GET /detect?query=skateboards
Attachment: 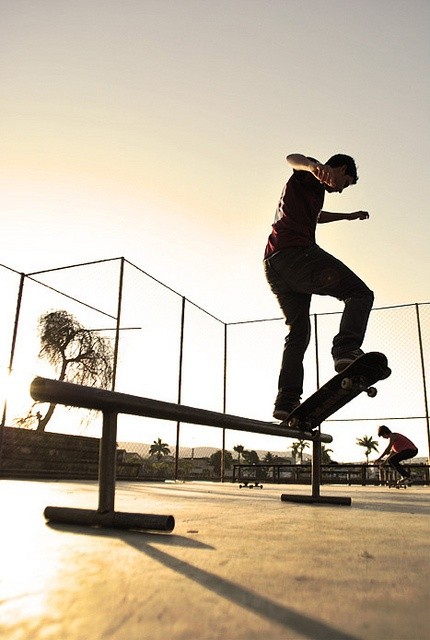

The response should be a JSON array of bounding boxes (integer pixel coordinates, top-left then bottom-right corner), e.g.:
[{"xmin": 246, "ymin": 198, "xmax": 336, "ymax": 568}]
[
  {"xmin": 388, "ymin": 481, "xmax": 412, "ymax": 487},
  {"xmin": 236, "ymin": 478, "xmax": 263, "ymax": 489},
  {"xmin": 280, "ymin": 351, "xmax": 391, "ymax": 441}
]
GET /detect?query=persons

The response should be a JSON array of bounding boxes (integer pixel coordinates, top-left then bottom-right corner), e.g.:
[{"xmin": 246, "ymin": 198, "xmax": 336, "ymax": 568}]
[
  {"xmin": 377, "ymin": 425, "xmax": 418, "ymax": 481},
  {"xmin": 264, "ymin": 154, "xmax": 375, "ymax": 420}
]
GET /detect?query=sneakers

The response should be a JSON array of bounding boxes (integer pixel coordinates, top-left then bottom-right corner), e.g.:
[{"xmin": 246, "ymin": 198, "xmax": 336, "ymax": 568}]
[
  {"xmin": 403, "ymin": 476, "xmax": 413, "ymax": 483},
  {"xmin": 396, "ymin": 475, "xmax": 407, "ymax": 483},
  {"xmin": 272, "ymin": 397, "xmax": 300, "ymax": 419},
  {"xmin": 333, "ymin": 348, "xmax": 363, "ymax": 372}
]
